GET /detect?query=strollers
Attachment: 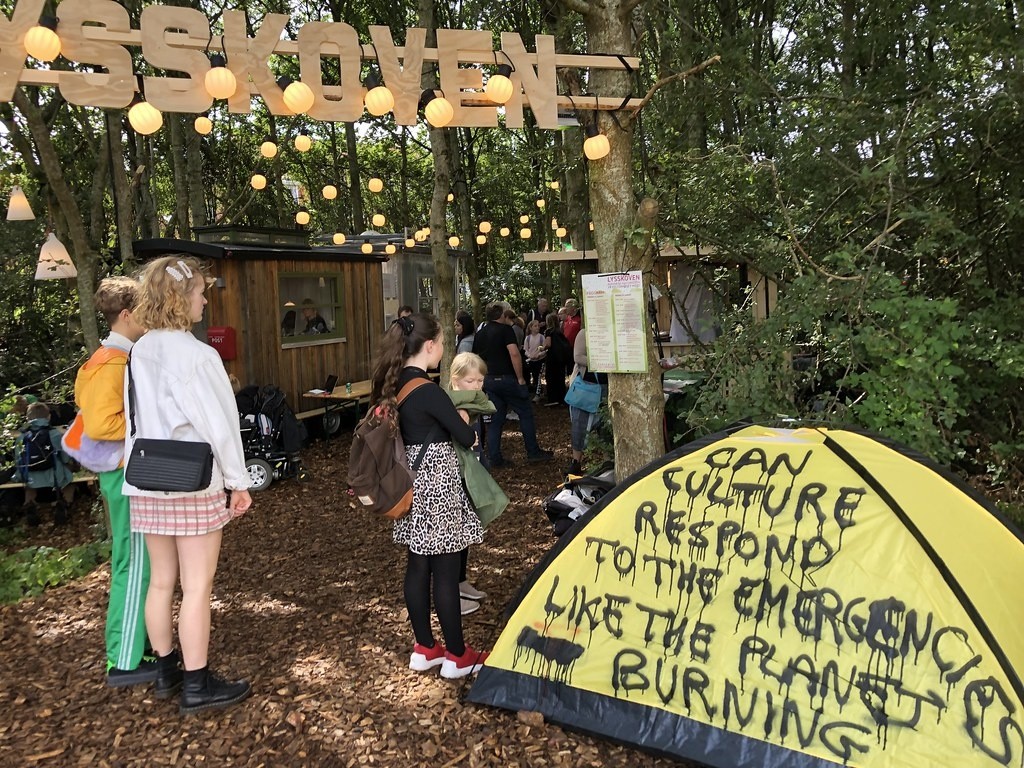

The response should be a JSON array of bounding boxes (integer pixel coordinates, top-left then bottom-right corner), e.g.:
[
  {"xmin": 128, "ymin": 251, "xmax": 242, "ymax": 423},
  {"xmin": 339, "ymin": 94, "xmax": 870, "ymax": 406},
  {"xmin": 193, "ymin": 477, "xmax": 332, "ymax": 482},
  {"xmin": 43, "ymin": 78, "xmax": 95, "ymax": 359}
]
[{"xmin": 233, "ymin": 384, "xmax": 305, "ymax": 492}]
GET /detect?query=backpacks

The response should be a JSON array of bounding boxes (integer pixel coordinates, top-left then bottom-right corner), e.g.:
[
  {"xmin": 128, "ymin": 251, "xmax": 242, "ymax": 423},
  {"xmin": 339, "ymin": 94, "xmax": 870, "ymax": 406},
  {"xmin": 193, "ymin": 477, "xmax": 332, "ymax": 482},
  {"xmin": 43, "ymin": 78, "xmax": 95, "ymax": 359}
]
[
  {"xmin": 346, "ymin": 377, "xmax": 436, "ymax": 520},
  {"xmin": 20, "ymin": 426, "xmax": 56, "ymax": 472},
  {"xmin": 551, "ymin": 332, "xmax": 573, "ymax": 362}
]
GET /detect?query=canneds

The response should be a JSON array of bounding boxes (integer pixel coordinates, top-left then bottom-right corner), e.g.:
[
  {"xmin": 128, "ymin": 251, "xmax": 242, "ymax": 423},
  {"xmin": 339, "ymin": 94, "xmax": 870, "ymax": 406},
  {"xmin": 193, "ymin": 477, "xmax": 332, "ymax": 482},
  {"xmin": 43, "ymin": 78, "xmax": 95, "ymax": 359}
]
[{"xmin": 346, "ymin": 383, "xmax": 351, "ymax": 394}]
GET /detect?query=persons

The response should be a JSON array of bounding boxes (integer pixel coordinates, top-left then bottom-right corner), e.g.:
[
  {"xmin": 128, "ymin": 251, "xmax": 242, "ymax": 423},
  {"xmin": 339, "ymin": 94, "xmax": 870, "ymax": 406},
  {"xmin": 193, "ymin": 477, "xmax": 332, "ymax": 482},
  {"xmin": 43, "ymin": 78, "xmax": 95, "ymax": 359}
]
[
  {"xmin": 455, "ymin": 297, "xmax": 583, "ymax": 423},
  {"xmin": 367, "ymin": 313, "xmax": 493, "ymax": 678},
  {"xmin": 568, "ymin": 328, "xmax": 608, "ymax": 476},
  {"xmin": 124, "ymin": 254, "xmax": 253, "ymax": 712},
  {"xmin": 282, "ymin": 298, "xmax": 331, "ymax": 338},
  {"xmin": 12, "ymin": 394, "xmax": 81, "ymax": 527},
  {"xmin": 445, "ymin": 349, "xmax": 489, "ymax": 616},
  {"xmin": 74, "ymin": 275, "xmax": 159, "ymax": 689},
  {"xmin": 398, "ymin": 306, "xmax": 414, "ymax": 319},
  {"xmin": 472, "ymin": 305, "xmax": 554, "ymax": 468}
]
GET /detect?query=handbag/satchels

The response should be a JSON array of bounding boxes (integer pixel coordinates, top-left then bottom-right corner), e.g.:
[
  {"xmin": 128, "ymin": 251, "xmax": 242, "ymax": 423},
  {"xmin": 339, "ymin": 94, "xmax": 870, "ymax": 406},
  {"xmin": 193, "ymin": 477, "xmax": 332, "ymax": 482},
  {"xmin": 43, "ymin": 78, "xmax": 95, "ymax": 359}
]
[
  {"xmin": 125, "ymin": 343, "xmax": 215, "ymax": 492},
  {"xmin": 61, "ymin": 409, "xmax": 127, "ymax": 474},
  {"xmin": 564, "ymin": 372, "xmax": 602, "ymax": 414}
]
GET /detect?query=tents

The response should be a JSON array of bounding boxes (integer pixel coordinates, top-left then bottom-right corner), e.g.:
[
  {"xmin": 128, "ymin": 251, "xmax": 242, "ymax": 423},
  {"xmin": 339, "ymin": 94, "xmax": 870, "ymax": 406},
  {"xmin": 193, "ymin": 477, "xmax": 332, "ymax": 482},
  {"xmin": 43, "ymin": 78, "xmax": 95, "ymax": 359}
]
[{"xmin": 460, "ymin": 417, "xmax": 1024, "ymax": 768}]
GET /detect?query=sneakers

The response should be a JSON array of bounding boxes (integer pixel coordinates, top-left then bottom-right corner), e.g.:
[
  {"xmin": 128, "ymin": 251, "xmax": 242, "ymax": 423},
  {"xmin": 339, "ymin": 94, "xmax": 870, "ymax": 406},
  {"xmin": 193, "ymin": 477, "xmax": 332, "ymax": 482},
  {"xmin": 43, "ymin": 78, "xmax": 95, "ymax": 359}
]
[
  {"xmin": 532, "ymin": 392, "xmax": 545, "ymax": 403},
  {"xmin": 409, "ymin": 639, "xmax": 445, "ymax": 672},
  {"xmin": 460, "ymin": 579, "xmax": 487, "ymax": 600},
  {"xmin": 440, "ymin": 645, "xmax": 491, "ymax": 679},
  {"xmin": 460, "ymin": 598, "xmax": 480, "ymax": 615},
  {"xmin": 109, "ymin": 656, "xmax": 182, "ymax": 687}
]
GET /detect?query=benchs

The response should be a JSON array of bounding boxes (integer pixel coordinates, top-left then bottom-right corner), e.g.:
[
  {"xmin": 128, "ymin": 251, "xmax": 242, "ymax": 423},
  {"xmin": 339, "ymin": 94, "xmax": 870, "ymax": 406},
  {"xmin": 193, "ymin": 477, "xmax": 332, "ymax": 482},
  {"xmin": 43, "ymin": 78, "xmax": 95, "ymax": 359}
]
[{"xmin": 0, "ymin": 471, "xmax": 98, "ymax": 504}]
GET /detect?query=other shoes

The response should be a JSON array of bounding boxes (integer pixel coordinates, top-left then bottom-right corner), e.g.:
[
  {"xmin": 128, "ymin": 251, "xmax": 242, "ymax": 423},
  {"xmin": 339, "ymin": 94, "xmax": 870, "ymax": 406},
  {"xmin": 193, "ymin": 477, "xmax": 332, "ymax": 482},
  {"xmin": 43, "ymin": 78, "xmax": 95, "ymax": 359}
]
[
  {"xmin": 488, "ymin": 458, "xmax": 515, "ymax": 468},
  {"xmin": 528, "ymin": 450, "xmax": 554, "ymax": 461}
]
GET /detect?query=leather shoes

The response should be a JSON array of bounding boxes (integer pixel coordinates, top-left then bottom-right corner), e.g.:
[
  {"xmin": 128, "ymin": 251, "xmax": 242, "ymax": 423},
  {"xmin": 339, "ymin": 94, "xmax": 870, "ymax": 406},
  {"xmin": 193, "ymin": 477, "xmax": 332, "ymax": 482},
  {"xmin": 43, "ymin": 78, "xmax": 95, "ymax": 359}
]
[
  {"xmin": 179, "ymin": 674, "xmax": 252, "ymax": 714},
  {"xmin": 156, "ymin": 666, "xmax": 183, "ymax": 700}
]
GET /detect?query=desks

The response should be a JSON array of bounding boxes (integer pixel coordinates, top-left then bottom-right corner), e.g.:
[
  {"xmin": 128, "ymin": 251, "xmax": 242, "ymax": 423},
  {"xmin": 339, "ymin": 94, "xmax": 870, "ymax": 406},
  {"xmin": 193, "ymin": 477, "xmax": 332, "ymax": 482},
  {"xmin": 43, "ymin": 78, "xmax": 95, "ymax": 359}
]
[{"xmin": 303, "ymin": 372, "xmax": 441, "ymax": 448}]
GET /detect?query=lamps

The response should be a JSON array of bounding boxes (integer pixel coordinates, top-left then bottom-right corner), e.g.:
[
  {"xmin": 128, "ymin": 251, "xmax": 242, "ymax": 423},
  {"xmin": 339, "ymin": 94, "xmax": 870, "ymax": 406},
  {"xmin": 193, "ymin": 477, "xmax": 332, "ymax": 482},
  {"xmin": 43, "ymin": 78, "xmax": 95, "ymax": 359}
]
[
  {"xmin": 6, "ymin": 119, "xmax": 37, "ymax": 221},
  {"xmin": 34, "ymin": 196, "xmax": 78, "ymax": 278}
]
